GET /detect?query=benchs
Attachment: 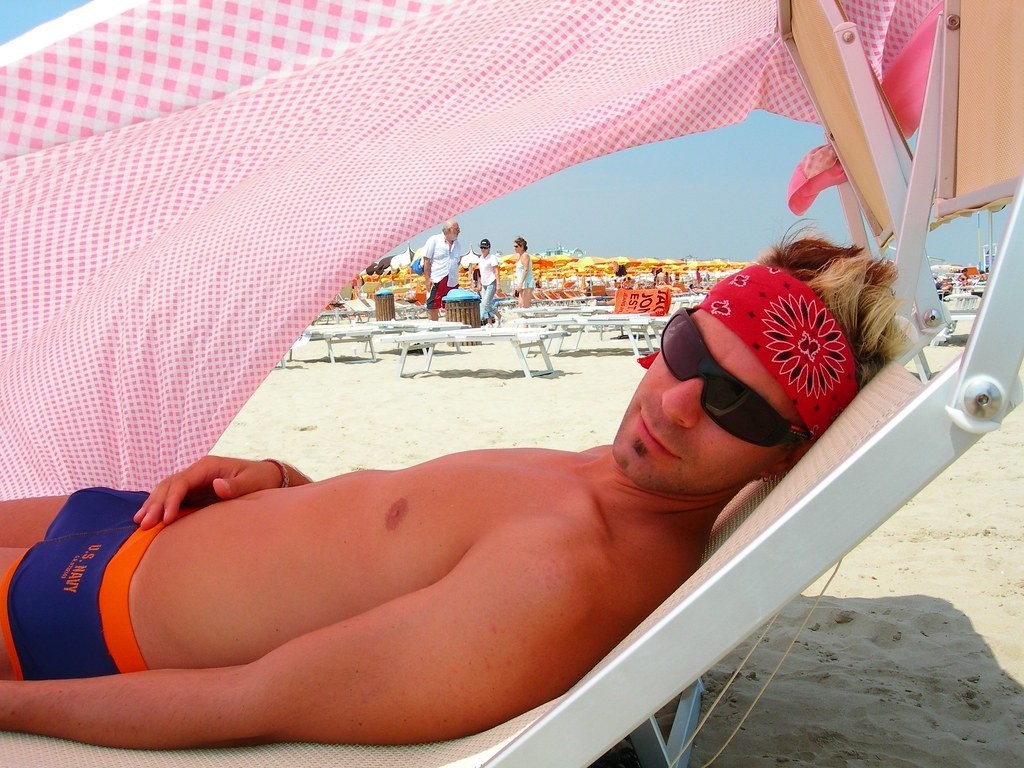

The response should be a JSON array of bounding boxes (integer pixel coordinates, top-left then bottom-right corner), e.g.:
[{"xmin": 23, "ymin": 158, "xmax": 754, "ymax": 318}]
[{"xmin": 305, "ymin": 295, "xmax": 709, "ymax": 379}]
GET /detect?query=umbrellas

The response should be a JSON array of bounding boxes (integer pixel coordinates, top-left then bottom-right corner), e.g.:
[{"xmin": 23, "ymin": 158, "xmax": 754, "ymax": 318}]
[{"xmin": 358, "ymin": 255, "xmax": 760, "ymax": 291}]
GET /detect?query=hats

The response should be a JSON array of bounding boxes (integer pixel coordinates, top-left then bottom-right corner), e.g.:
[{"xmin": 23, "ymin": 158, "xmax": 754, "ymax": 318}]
[{"xmin": 480, "ymin": 239, "xmax": 490, "ymax": 247}]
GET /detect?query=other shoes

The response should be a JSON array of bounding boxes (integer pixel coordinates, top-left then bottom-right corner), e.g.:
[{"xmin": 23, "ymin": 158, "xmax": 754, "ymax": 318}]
[
  {"xmin": 481, "ymin": 323, "xmax": 493, "ymax": 328},
  {"xmin": 496, "ymin": 317, "xmax": 504, "ymax": 328}
]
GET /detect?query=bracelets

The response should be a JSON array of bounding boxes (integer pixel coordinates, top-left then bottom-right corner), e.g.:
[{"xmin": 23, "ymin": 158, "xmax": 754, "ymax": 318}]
[{"xmin": 259, "ymin": 458, "xmax": 289, "ymax": 488}]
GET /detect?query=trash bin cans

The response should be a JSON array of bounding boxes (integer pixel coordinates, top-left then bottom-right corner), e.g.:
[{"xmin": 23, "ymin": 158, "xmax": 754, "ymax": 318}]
[
  {"xmin": 442, "ymin": 289, "xmax": 483, "ymax": 347},
  {"xmin": 373, "ymin": 289, "xmax": 395, "ymax": 322}
]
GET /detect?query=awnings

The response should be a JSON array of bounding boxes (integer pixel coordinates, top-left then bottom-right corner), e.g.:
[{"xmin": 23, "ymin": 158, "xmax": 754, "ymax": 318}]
[{"xmin": 0, "ymin": 0, "xmax": 942, "ymax": 494}]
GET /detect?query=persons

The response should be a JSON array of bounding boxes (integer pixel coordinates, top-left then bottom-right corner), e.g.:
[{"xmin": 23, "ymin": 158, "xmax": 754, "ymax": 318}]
[
  {"xmin": 615, "ymin": 265, "xmax": 622, "ymax": 277},
  {"xmin": 650, "ymin": 268, "xmax": 656, "ymax": 276},
  {"xmin": 656, "ymin": 268, "xmax": 666, "ymax": 286},
  {"xmin": 695, "ymin": 268, "xmax": 703, "ymax": 284},
  {"xmin": 422, "ymin": 219, "xmax": 464, "ymax": 321},
  {"xmin": 613, "ymin": 280, "xmax": 621, "ymax": 289},
  {"xmin": 689, "ymin": 284, "xmax": 714, "ymax": 294},
  {"xmin": 933, "ymin": 268, "xmax": 988, "ymax": 303},
  {"xmin": 664, "ymin": 272, "xmax": 673, "ymax": 285},
  {"xmin": 621, "ymin": 278, "xmax": 629, "ymax": 290},
  {"xmin": 0, "ymin": 217, "xmax": 919, "ymax": 751},
  {"xmin": 587, "ymin": 281, "xmax": 593, "ymax": 294},
  {"xmin": 654, "ymin": 270, "xmax": 661, "ymax": 282},
  {"xmin": 470, "ymin": 238, "xmax": 507, "ymax": 331},
  {"xmin": 513, "ymin": 235, "xmax": 537, "ymax": 329},
  {"xmin": 613, "ymin": 261, "xmax": 619, "ymax": 273},
  {"xmin": 622, "ymin": 265, "xmax": 628, "ymax": 275},
  {"xmin": 627, "ymin": 277, "xmax": 635, "ymax": 290}
]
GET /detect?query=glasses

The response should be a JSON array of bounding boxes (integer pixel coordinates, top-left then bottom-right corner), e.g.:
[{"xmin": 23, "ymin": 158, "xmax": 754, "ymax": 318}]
[
  {"xmin": 450, "ymin": 227, "xmax": 460, "ymax": 233},
  {"xmin": 480, "ymin": 247, "xmax": 489, "ymax": 250},
  {"xmin": 658, "ymin": 306, "xmax": 814, "ymax": 448},
  {"xmin": 514, "ymin": 245, "xmax": 519, "ymax": 248}
]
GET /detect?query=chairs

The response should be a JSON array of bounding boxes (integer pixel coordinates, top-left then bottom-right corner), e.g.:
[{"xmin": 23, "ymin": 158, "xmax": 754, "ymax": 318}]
[
  {"xmin": 0, "ymin": 1, "xmax": 1024, "ymax": 768},
  {"xmin": 296, "ymin": 282, "xmax": 687, "ymax": 308}
]
